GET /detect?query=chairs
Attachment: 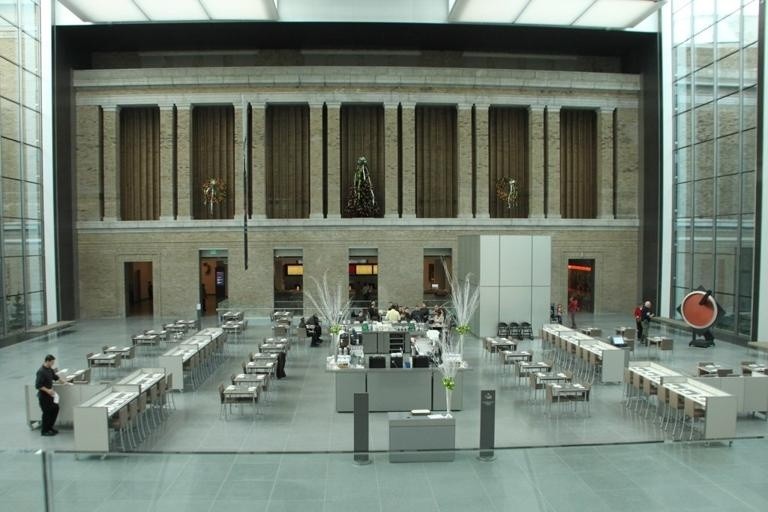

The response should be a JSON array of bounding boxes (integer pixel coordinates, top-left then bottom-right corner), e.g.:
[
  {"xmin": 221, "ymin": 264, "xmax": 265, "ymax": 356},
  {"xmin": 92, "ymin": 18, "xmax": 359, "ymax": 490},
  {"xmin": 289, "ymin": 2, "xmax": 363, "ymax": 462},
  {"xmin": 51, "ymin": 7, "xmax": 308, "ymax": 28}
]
[
  {"xmin": 28, "ymin": 309, "xmax": 293, "ymax": 459},
  {"xmin": 483, "ymin": 321, "xmax": 768, "ymax": 444}
]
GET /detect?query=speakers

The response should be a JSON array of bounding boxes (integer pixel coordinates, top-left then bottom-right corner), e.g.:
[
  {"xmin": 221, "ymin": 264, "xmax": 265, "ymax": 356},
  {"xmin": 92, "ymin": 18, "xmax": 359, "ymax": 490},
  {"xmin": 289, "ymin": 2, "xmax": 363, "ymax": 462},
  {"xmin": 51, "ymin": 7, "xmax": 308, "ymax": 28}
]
[
  {"xmin": 352, "ymin": 392, "xmax": 371, "ymax": 465},
  {"xmin": 477, "ymin": 389, "xmax": 496, "ymax": 461}
]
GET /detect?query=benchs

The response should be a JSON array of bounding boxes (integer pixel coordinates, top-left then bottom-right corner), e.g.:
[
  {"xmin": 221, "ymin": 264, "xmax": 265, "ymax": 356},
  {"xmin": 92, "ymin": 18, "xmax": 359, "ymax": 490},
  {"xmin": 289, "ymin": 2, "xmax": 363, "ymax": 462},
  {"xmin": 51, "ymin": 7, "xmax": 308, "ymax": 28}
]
[{"xmin": 297, "ymin": 317, "xmax": 323, "ymax": 341}]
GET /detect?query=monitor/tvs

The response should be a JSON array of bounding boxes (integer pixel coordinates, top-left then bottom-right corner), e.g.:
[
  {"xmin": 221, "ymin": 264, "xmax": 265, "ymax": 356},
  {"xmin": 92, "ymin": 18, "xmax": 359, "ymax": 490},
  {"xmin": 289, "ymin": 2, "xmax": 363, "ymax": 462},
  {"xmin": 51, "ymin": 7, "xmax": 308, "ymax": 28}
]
[
  {"xmin": 369, "ymin": 356, "xmax": 386, "ymax": 367},
  {"xmin": 412, "ymin": 355, "xmax": 429, "ymax": 367}
]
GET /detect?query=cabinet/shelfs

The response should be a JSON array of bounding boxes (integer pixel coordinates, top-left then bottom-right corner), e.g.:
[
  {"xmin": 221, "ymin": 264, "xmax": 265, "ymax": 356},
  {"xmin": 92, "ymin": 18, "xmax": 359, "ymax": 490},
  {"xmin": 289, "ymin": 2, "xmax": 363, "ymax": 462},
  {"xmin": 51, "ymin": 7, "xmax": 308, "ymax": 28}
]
[{"xmin": 388, "ymin": 412, "xmax": 455, "ymax": 461}]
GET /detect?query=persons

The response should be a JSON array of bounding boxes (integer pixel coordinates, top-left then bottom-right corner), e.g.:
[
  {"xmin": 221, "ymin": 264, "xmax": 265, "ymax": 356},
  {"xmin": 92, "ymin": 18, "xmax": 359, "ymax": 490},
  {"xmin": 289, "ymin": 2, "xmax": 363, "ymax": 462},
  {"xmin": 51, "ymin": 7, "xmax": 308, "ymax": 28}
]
[
  {"xmin": 298, "ymin": 313, "xmax": 323, "ymax": 346},
  {"xmin": 35, "ymin": 354, "xmax": 68, "ymax": 435},
  {"xmin": 633, "ymin": 301, "xmax": 655, "ymax": 345},
  {"xmin": 569, "ymin": 294, "xmax": 579, "ymax": 328},
  {"xmin": 352, "ymin": 300, "xmax": 446, "ymax": 324}
]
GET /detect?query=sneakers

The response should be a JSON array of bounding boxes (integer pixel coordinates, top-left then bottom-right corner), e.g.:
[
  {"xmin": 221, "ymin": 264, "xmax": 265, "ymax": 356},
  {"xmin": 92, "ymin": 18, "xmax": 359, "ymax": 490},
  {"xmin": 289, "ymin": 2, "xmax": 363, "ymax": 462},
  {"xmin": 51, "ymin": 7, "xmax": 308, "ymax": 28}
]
[{"xmin": 41, "ymin": 428, "xmax": 58, "ymax": 436}]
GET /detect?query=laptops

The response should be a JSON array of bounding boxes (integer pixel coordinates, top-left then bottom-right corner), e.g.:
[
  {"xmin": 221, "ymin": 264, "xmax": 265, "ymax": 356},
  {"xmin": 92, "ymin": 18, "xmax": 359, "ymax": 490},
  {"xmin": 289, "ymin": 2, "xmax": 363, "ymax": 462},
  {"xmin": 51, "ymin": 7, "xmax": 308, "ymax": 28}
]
[{"xmin": 612, "ymin": 335, "xmax": 628, "ymax": 347}]
